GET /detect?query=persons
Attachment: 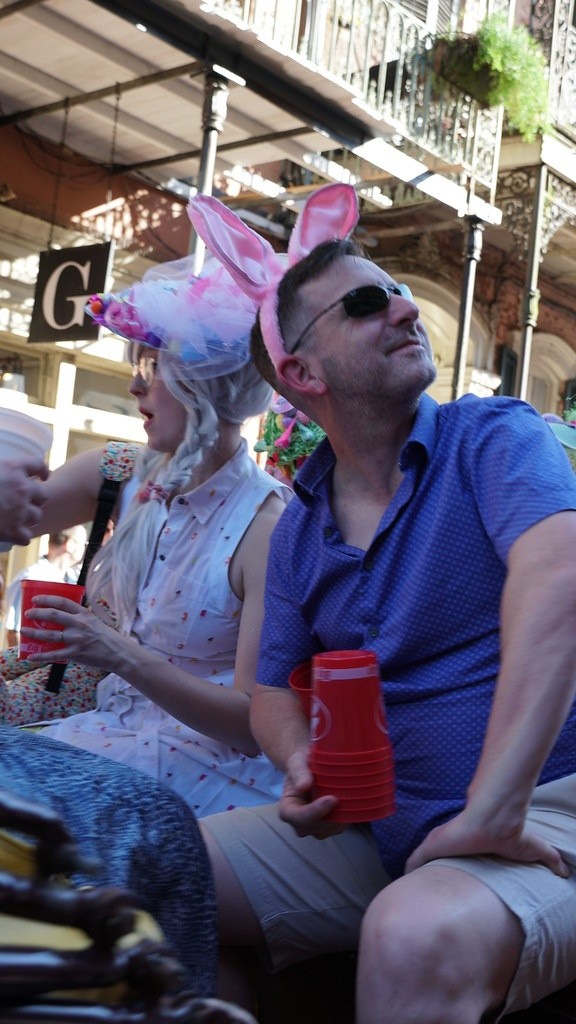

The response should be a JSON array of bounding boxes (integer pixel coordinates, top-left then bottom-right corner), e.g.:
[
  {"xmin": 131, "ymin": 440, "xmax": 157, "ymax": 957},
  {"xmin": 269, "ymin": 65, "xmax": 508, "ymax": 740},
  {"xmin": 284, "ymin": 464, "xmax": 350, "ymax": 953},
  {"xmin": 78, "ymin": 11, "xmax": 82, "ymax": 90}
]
[
  {"xmin": 6, "ymin": 525, "xmax": 88, "ymax": 651},
  {"xmin": 194, "ymin": 240, "xmax": 575, "ymax": 1024},
  {"xmin": 0, "ymin": 440, "xmax": 221, "ymax": 1004},
  {"xmin": 0, "ymin": 257, "xmax": 298, "ymax": 818}
]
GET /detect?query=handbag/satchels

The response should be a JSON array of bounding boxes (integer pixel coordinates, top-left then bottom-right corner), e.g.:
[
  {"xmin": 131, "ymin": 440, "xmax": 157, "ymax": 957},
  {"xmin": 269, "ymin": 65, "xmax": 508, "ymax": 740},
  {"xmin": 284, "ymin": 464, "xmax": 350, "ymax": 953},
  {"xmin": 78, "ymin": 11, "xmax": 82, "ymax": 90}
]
[{"xmin": 0, "ymin": 597, "xmax": 118, "ymax": 727}]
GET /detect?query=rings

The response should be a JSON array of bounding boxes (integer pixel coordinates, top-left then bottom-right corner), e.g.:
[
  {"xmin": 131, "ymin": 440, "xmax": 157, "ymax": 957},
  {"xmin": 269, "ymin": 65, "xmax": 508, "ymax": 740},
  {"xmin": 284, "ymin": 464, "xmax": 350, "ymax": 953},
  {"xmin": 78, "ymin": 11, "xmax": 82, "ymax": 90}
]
[{"xmin": 61, "ymin": 631, "xmax": 63, "ymax": 641}]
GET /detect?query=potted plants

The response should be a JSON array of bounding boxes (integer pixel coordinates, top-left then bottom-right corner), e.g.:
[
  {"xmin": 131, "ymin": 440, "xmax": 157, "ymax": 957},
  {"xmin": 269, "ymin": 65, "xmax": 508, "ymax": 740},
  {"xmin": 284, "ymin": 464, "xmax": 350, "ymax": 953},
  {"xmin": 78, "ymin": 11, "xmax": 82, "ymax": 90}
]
[{"xmin": 425, "ymin": 11, "xmax": 551, "ymax": 143}]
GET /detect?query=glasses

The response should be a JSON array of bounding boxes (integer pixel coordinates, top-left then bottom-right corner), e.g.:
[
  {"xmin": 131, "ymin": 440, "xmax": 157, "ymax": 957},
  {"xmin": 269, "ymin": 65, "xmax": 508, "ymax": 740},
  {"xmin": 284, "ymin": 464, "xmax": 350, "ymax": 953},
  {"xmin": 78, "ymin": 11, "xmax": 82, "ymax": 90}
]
[
  {"xmin": 288, "ymin": 282, "xmax": 414, "ymax": 354},
  {"xmin": 130, "ymin": 353, "xmax": 173, "ymax": 383}
]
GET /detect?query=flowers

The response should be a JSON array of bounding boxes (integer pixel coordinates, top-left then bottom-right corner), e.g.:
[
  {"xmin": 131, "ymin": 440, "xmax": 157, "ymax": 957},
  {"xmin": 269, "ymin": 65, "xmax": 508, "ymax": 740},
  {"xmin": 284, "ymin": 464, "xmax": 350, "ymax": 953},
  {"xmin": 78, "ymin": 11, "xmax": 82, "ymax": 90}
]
[{"xmin": 87, "ymin": 293, "xmax": 147, "ymax": 347}]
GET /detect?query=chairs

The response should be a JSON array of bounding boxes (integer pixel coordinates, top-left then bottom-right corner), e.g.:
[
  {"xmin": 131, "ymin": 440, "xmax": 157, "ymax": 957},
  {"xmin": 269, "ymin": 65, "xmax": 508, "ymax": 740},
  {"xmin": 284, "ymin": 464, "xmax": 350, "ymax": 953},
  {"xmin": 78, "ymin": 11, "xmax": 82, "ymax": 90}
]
[{"xmin": 0, "ymin": 792, "xmax": 189, "ymax": 1024}]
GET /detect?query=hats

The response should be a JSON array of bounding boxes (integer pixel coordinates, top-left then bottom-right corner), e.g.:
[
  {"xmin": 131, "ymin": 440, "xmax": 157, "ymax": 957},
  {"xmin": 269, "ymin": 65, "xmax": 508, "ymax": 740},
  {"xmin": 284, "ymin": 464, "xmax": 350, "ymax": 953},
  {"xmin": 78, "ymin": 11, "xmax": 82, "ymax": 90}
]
[{"xmin": 82, "ymin": 246, "xmax": 271, "ymax": 363}]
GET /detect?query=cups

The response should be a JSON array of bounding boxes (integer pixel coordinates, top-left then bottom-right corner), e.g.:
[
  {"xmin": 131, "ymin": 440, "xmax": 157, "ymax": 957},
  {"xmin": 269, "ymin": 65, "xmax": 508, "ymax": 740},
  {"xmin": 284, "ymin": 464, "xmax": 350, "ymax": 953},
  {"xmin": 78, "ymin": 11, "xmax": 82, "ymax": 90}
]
[
  {"xmin": 17, "ymin": 578, "xmax": 88, "ymax": 664},
  {"xmin": 288, "ymin": 649, "xmax": 397, "ymax": 822},
  {"xmin": 0, "ymin": 408, "xmax": 51, "ymax": 552}
]
